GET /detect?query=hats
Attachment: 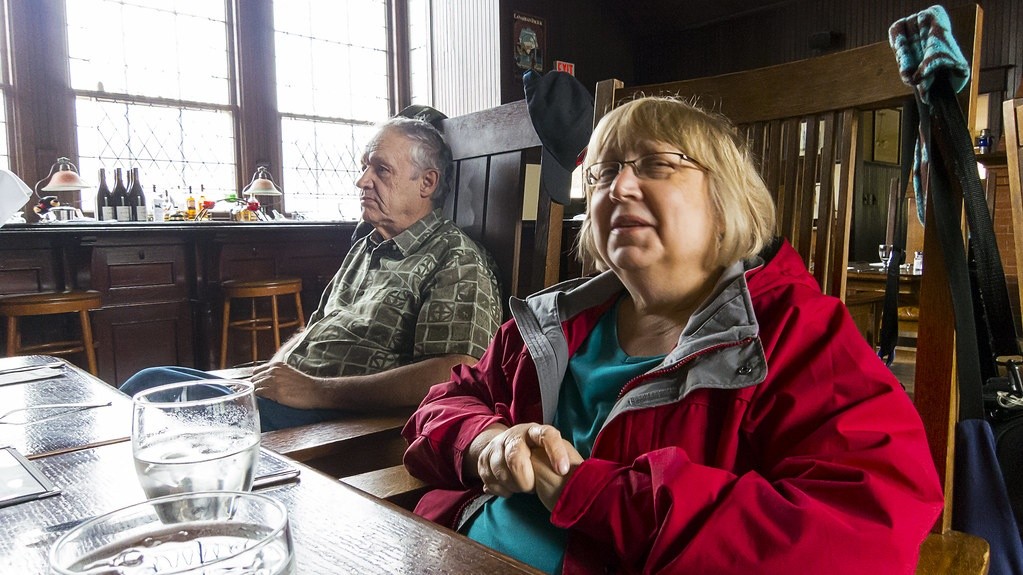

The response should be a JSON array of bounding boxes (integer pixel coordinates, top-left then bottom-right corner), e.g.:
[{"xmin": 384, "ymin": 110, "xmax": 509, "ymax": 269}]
[{"xmin": 524, "ymin": 68, "xmax": 595, "ymax": 207}]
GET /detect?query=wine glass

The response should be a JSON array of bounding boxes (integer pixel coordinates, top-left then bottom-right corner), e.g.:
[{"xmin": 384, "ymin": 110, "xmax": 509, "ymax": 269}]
[{"xmin": 878, "ymin": 245, "xmax": 893, "ymax": 272}]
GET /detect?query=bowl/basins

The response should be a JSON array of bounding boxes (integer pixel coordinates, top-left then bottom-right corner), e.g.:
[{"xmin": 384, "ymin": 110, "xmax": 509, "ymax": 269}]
[
  {"xmin": 51, "ymin": 206, "xmax": 76, "ymax": 220},
  {"xmin": 49, "ymin": 492, "xmax": 298, "ymax": 575}
]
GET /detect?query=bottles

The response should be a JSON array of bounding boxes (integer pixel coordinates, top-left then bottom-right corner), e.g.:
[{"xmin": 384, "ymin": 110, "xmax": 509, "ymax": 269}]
[
  {"xmin": 913, "ymin": 251, "xmax": 923, "ymax": 274},
  {"xmin": 95, "ymin": 168, "xmax": 186, "ymax": 221},
  {"xmin": 186, "ymin": 186, "xmax": 197, "ymax": 220},
  {"xmin": 198, "ymin": 184, "xmax": 208, "ymax": 221},
  {"xmin": 248, "ymin": 194, "xmax": 259, "ymax": 220},
  {"xmin": 978, "ymin": 129, "xmax": 989, "ymax": 155}
]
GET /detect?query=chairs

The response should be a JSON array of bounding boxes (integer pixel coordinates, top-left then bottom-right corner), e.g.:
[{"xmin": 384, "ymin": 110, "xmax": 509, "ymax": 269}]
[{"xmin": 207, "ymin": 3, "xmax": 993, "ymax": 575}]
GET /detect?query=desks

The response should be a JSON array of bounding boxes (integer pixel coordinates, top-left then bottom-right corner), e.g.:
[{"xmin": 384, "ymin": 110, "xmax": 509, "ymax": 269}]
[
  {"xmin": 0, "ymin": 356, "xmax": 543, "ymax": 575},
  {"xmin": 847, "ymin": 261, "xmax": 924, "ymax": 344}
]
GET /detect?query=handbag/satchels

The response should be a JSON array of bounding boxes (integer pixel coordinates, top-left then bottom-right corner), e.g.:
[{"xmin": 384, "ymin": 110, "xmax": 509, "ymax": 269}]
[{"xmin": 953, "ymin": 396, "xmax": 1023, "ymax": 575}]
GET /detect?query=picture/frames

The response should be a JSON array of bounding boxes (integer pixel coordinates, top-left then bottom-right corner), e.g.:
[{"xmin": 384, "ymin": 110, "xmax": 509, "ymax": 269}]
[
  {"xmin": 873, "ymin": 107, "xmax": 903, "ymax": 167},
  {"xmin": 511, "ymin": 10, "xmax": 547, "ymax": 74}
]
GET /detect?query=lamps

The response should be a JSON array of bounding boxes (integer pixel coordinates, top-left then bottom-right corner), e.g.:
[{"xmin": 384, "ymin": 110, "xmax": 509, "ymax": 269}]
[
  {"xmin": 34, "ymin": 157, "xmax": 94, "ymax": 222},
  {"xmin": 241, "ymin": 166, "xmax": 283, "ymax": 201}
]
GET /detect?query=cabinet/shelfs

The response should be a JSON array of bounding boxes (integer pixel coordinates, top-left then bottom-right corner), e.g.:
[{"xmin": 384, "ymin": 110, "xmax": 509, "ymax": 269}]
[{"xmin": 0, "ymin": 220, "xmax": 359, "ymax": 388}]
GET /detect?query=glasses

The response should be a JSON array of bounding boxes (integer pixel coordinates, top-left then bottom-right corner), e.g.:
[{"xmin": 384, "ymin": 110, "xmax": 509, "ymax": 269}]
[{"xmin": 586, "ymin": 151, "xmax": 714, "ymax": 187}]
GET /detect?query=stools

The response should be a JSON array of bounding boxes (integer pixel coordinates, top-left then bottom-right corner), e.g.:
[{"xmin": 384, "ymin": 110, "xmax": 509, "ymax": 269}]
[
  {"xmin": 0, "ymin": 288, "xmax": 102, "ymax": 377},
  {"xmin": 219, "ymin": 274, "xmax": 304, "ymax": 369}
]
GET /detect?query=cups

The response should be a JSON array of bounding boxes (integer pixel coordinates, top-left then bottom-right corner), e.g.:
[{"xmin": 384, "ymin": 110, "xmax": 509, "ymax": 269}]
[{"xmin": 131, "ymin": 380, "xmax": 261, "ymax": 522}]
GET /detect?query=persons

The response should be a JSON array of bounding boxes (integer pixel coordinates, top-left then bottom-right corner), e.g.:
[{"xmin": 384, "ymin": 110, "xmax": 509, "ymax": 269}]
[
  {"xmin": 118, "ymin": 117, "xmax": 503, "ymax": 436},
  {"xmin": 402, "ymin": 99, "xmax": 945, "ymax": 575}
]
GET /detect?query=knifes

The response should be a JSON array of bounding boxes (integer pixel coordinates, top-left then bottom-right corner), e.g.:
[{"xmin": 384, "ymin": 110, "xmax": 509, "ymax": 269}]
[{"xmin": 46, "ymin": 469, "xmax": 300, "ymax": 533}]
